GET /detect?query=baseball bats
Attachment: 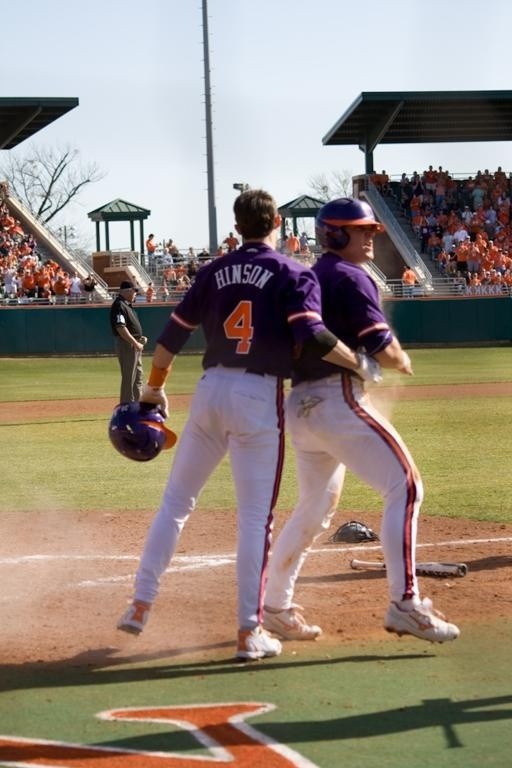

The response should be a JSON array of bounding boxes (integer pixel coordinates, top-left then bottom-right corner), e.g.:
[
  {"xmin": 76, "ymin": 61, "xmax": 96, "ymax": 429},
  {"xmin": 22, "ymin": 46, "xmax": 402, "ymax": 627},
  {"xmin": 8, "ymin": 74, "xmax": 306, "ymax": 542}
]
[{"xmin": 349, "ymin": 558, "xmax": 468, "ymax": 578}]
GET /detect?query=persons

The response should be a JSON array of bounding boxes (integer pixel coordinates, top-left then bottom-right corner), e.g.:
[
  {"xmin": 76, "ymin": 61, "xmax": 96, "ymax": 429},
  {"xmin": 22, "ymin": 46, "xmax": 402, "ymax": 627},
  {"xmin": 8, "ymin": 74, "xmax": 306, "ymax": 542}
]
[
  {"xmin": 372, "ymin": 165, "xmax": 512, "ymax": 297},
  {"xmin": 111, "ymin": 281, "xmax": 147, "ymax": 404},
  {"xmin": 275, "ymin": 232, "xmax": 310, "ymax": 258},
  {"xmin": 117, "ymin": 190, "xmax": 383, "ymax": 662},
  {"xmin": 0, "ymin": 165, "xmax": 239, "ymax": 303},
  {"xmin": 261, "ymin": 198, "xmax": 460, "ymax": 643}
]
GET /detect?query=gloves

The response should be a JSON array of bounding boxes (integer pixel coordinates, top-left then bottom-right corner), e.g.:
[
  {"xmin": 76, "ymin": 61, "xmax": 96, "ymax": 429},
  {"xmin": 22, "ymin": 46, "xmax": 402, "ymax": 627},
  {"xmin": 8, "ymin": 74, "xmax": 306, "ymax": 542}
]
[
  {"xmin": 354, "ymin": 353, "xmax": 384, "ymax": 383},
  {"xmin": 139, "ymin": 382, "xmax": 169, "ymax": 417}
]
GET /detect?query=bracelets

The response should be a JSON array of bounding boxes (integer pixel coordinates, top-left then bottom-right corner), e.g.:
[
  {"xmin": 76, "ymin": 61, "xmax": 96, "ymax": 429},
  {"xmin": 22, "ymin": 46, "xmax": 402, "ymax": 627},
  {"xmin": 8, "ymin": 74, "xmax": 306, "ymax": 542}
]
[{"xmin": 147, "ymin": 366, "xmax": 169, "ymax": 386}]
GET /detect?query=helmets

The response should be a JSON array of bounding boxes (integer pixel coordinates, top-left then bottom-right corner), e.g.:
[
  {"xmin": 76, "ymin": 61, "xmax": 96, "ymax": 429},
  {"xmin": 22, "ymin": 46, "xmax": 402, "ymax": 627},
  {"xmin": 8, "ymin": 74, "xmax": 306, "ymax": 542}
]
[
  {"xmin": 315, "ymin": 197, "xmax": 385, "ymax": 251},
  {"xmin": 109, "ymin": 401, "xmax": 177, "ymax": 463}
]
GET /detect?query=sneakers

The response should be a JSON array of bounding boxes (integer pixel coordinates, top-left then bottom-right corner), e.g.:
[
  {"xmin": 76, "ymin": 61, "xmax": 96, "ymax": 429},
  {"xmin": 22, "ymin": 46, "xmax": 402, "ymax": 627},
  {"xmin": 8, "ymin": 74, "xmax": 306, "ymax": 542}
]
[
  {"xmin": 383, "ymin": 597, "xmax": 461, "ymax": 643},
  {"xmin": 117, "ymin": 603, "xmax": 150, "ymax": 635},
  {"xmin": 237, "ymin": 625, "xmax": 282, "ymax": 660},
  {"xmin": 261, "ymin": 604, "xmax": 322, "ymax": 640}
]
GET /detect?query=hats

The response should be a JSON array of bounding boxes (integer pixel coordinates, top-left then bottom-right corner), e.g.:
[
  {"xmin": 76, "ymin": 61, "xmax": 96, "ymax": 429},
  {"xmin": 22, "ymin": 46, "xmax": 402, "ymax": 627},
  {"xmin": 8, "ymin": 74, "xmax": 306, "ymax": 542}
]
[{"xmin": 120, "ymin": 281, "xmax": 135, "ymax": 289}]
